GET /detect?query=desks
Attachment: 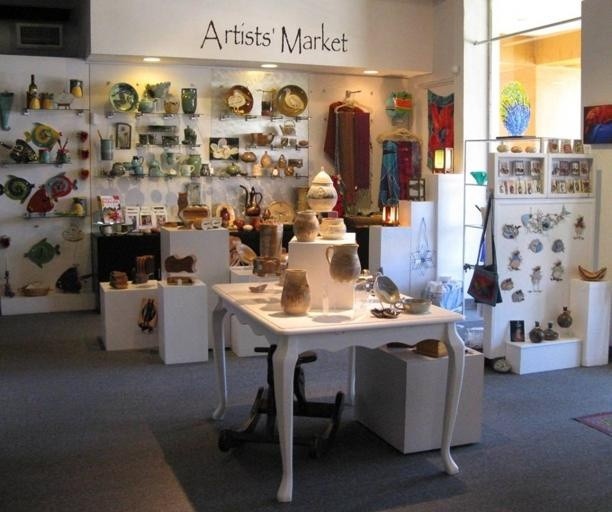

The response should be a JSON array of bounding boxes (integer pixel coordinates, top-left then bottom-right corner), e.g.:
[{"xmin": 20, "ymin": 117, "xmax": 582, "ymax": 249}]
[{"xmin": 210, "ymin": 280, "xmax": 467, "ymax": 504}]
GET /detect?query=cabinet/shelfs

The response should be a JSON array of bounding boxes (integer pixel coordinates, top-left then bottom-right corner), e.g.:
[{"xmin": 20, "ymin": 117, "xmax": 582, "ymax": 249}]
[{"xmin": 461, "ymin": 135, "xmax": 561, "ymax": 349}]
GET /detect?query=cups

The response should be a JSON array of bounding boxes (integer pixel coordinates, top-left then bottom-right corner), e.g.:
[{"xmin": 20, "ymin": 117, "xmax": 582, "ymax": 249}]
[
  {"xmin": 261, "ymin": 89, "xmax": 277, "ymax": 117},
  {"xmin": 101, "ymin": 139, "xmax": 113, "ymax": 160},
  {"xmin": 164, "ymin": 88, "xmax": 196, "ymax": 113},
  {"xmin": 180, "ymin": 165, "xmax": 194, "ymax": 177}
]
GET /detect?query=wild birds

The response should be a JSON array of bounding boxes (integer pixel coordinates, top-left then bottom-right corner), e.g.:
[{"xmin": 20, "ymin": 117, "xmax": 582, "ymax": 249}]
[
  {"xmin": 373, "ymin": 265, "xmax": 404, "ymax": 313},
  {"xmin": 227, "ymin": 89, "xmax": 250, "ymax": 114},
  {"xmin": 278, "ymin": 88, "xmax": 305, "ymax": 111}
]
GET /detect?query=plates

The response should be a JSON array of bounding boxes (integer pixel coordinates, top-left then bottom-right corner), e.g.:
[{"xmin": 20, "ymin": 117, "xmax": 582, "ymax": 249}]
[
  {"xmin": 277, "ymin": 84, "xmax": 308, "ymax": 116},
  {"xmin": 108, "ymin": 82, "xmax": 138, "ymax": 113},
  {"xmin": 224, "ymin": 85, "xmax": 252, "ymax": 115}
]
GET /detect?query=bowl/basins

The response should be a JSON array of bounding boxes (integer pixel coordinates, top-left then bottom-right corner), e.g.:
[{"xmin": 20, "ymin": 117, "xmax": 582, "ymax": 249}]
[
  {"xmin": 373, "ymin": 276, "xmax": 431, "ymax": 314},
  {"xmin": 17, "ymin": 287, "xmax": 51, "ymax": 295}
]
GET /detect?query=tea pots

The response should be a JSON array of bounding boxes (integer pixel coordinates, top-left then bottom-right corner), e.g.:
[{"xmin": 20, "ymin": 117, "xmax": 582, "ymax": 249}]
[
  {"xmin": 138, "ymin": 93, "xmax": 159, "ymax": 112},
  {"xmin": 278, "ymin": 120, "xmax": 297, "ymax": 137}
]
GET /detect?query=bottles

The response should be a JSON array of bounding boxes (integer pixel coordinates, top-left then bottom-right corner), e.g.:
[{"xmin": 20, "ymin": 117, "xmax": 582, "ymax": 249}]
[
  {"xmin": 529, "ymin": 306, "xmax": 572, "ymax": 344},
  {"xmin": 188, "ymin": 132, "xmax": 361, "ymax": 316},
  {"xmin": 29, "ymin": 73, "xmax": 37, "ymax": 101}
]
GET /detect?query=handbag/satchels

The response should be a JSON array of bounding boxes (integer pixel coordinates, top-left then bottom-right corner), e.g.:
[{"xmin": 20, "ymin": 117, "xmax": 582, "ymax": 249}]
[{"xmin": 467, "ymin": 264, "xmax": 503, "ymax": 307}]
